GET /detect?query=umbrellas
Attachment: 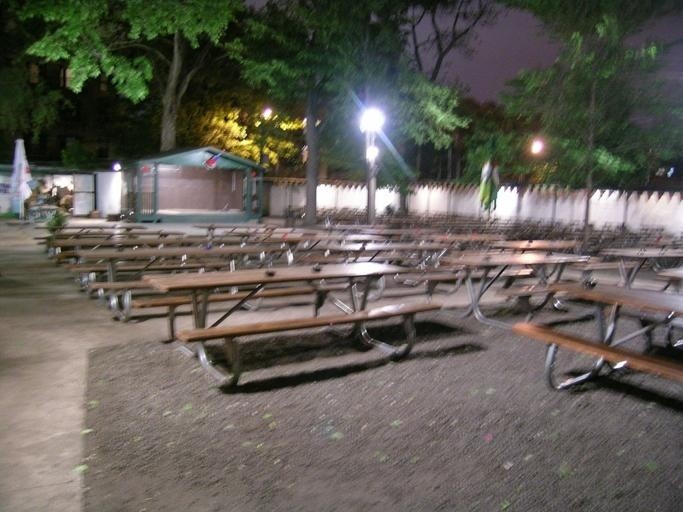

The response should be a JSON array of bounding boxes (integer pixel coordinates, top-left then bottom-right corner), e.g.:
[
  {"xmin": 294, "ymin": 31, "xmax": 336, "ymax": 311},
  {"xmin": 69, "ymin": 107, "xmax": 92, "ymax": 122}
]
[
  {"xmin": 11, "ymin": 139, "xmax": 32, "ymax": 220},
  {"xmin": 480, "ymin": 155, "xmax": 499, "ymax": 223}
]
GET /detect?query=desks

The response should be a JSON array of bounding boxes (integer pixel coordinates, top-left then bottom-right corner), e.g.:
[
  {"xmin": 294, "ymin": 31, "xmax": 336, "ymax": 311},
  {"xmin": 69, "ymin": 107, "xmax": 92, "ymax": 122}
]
[{"xmin": 31, "ymin": 206, "xmax": 682, "ymax": 392}]
